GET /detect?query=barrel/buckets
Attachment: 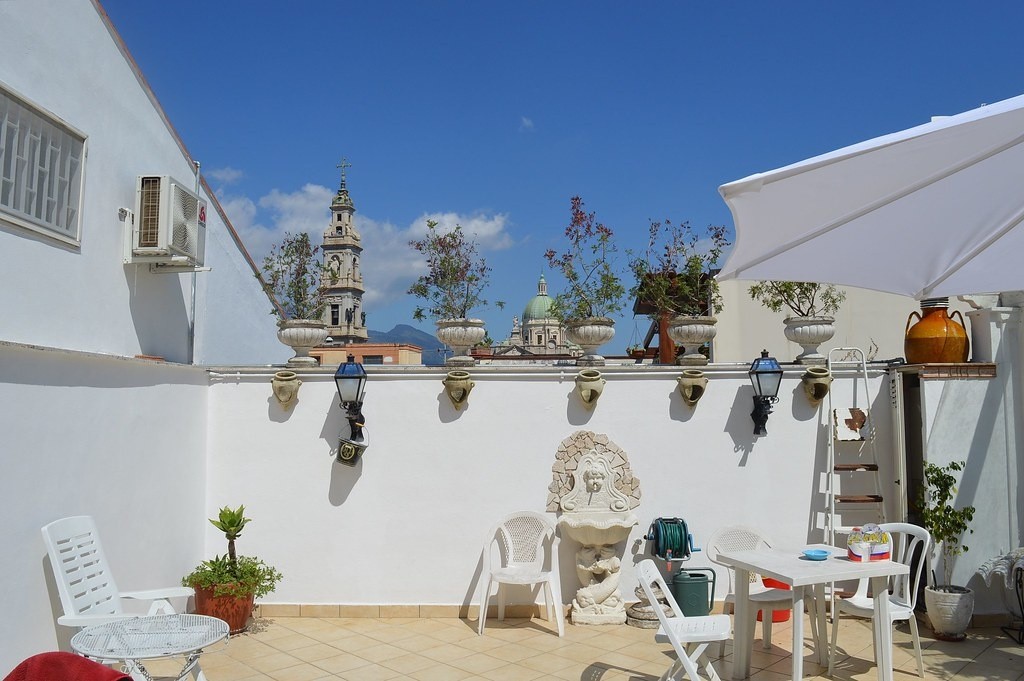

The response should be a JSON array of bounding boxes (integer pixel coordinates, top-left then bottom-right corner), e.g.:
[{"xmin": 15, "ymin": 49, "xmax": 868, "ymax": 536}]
[
  {"xmin": 756, "ymin": 575, "xmax": 792, "ymax": 624},
  {"xmin": 337, "ymin": 423, "xmax": 370, "ymax": 467}
]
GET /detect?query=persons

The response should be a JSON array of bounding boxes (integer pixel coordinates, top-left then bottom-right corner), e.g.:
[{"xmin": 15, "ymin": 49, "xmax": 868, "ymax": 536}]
[
  {"xmin": 574, "ymin": 547, "xmax": 621, "ymax": 606},
  {"xmin": 583, "ymin": 465, "xmax": 606, "ymax": 490}
]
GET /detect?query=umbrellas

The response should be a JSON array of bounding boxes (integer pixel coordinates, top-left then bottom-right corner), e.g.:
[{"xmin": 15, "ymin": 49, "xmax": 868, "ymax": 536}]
[{"xmin": 715, "ymin": 92, "xmax": 1024, "ymax": 298}]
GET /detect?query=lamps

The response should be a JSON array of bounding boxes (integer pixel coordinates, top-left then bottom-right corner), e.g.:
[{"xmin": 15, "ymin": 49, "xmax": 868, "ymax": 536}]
[
  {"xmin": 333, "ymin": 352, "xmax": 366, "ymax": 442},
  {"xmin": 750, "ymin": 349, "xmax": 783, "ymax": 436}
]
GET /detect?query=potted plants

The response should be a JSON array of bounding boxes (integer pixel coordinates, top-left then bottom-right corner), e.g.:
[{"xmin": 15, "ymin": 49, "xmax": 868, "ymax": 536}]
[
  {"xmin": 626, "ymin": 220, "xmax": 730, "ymax": 360},
  {"xmin": 748, "ymin": 279, "xmax": 844, "ymax": 358},
  {"xmin": 253, "ymin": 233, "xmax": 340, "ymax": 364},
  {"xmin": 180, "ymin": 503, "xmax": 284, "ymax": 636},
  {"xmin": 542, "ymin": 193, "xmax": 628, "ymax": 360},
  {"xmin": 405, "ymin": 219, "xmax": 487, "ymax": 363},
  {"xmin": 916, "ymin": 454, "xmax": 975, "ymax": 635}
]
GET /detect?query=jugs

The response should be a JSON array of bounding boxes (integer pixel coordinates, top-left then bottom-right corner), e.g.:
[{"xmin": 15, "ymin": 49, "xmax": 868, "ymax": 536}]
[{"xmin": 665, "ymin": 566, "xmax": 716, "ymax": 616}]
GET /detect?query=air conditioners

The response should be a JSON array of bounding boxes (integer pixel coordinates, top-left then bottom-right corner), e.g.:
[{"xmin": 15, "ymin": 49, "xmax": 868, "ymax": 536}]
[{"xmin": 131, "ymin": 175, "xmax": 207, "ymax": 269}]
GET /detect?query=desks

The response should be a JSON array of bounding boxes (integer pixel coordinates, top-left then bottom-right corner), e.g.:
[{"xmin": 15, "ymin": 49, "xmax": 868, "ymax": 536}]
[
  {"xmin": 717, "ymin": 543, "xmax": 910, "ymax": 681},
  {"xmin": 70, "ymin": 614, "xmax": 230, "ymax": 681}
]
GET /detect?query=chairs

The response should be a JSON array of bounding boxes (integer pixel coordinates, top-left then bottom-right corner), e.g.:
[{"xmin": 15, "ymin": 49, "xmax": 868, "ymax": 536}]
[
  {"xmin": 40, "ymin": 515, "xmax": 208, "ymax": 681},
  {"xmin": 478, "ymin": 511, "xmax": 564, "ymax": 637},
  {"xmin": 706, "ymin": 525, "xmax": 821, "ymax": 665},
  {"xmin": 828, "ymin": 523, "xmax": 931, "ymax": 678},
  {"xmin": 636, "ymin": 559, "xmax": 731, "ymax": 681}
]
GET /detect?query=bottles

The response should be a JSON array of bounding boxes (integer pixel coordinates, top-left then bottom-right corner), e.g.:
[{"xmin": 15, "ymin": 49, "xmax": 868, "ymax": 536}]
[{"xmin": 904, "ymin": 297, "xmax": 970, "ymax": 365}]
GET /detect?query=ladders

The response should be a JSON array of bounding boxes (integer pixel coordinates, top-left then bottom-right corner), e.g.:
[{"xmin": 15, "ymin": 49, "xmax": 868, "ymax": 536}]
[{"xmin": 828, "ymin": 347, "xmax": 895, "ymax": 624}]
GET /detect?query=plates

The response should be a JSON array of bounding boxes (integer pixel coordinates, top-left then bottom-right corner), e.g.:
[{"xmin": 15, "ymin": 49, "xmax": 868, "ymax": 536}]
[{"xmin": 802, "ymin": 550, "xmax": 831, "ymax": 560}]
